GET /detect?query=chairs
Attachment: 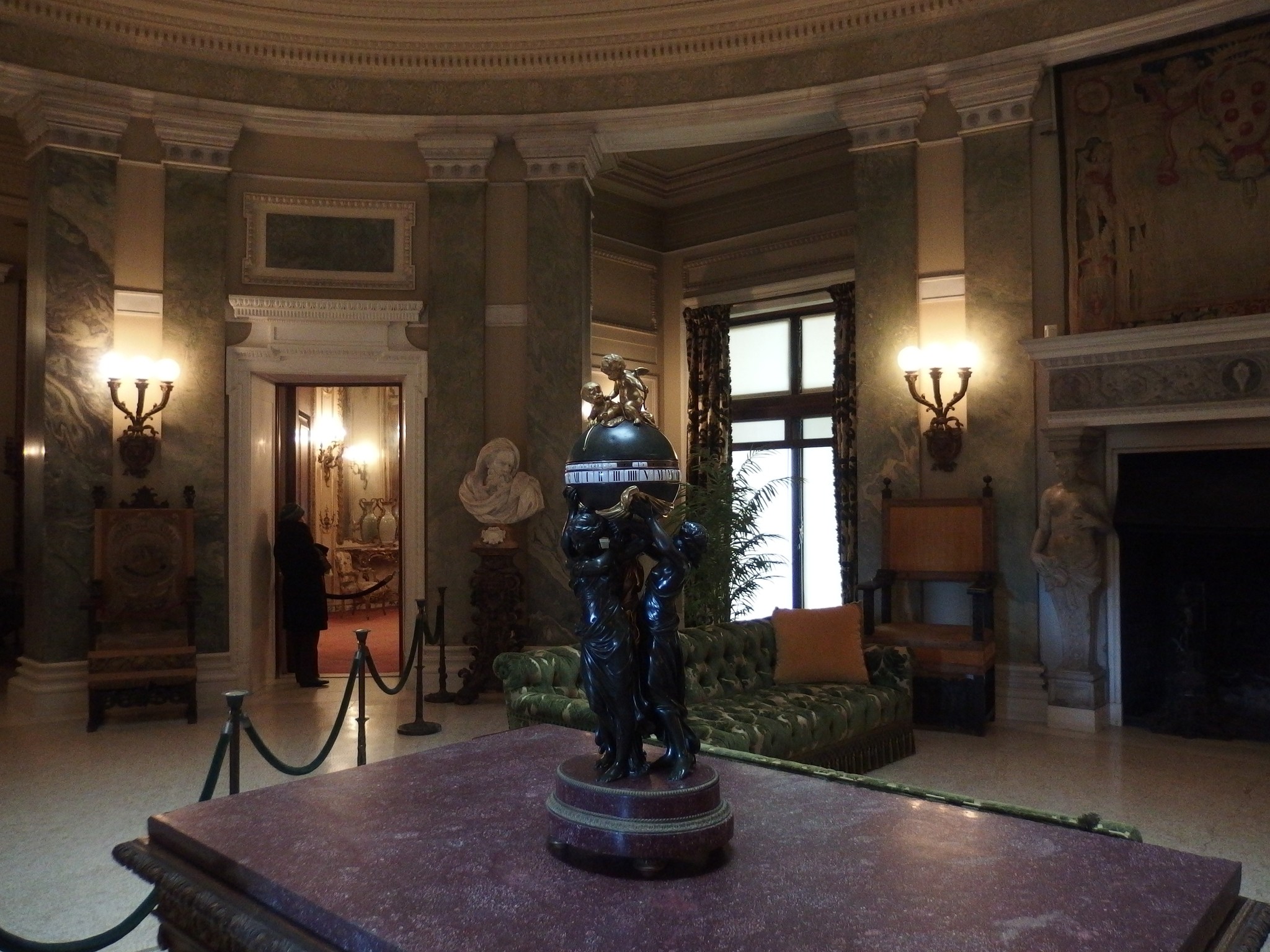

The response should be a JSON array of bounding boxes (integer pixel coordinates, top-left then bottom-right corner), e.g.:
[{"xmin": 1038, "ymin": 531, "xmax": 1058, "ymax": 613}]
[
  {"xmin": 856, "ymin": 475, "xmax": 1001, "ymax": 738},
  {"xmin": 333, "ymin": 547, "xmax": 390, "ymax": 620},
  {"xmin": 88, "ymin": 481, "xmax": 199, "ymax": 732}
]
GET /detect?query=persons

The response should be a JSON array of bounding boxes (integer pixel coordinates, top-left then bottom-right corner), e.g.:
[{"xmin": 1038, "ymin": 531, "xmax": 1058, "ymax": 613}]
[
  {"xmin": 273, "ymin": 504, "xmax": 331, "ymax": 687},
  {"xmin": 580, "ymin": 382, "xmax": 620, "ymax": 428},
  {"xmin": 600, "ymin": 353, "xmax": 650, "ymax": 425},
  {"xmin": 1029, "ymin": 454, "xmax": 1116, "ymax": 673},
  {"xmin": 459, "ymin": 437, "xmax": 546, "ymax": 525},
  {"xmin": 561, "ymin": 491, "xmax": 639, "ymax": 783},
  {"xmin": 631, "ymin": 494, "xmax": 708, "ymax": 779},
  {"xmin": 611, "ymin": 527, "xmax": 654, "ymax": 746}
]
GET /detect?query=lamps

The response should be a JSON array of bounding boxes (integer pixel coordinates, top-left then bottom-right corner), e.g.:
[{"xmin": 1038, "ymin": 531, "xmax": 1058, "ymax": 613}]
[
  {"xmin": 311, "ymin": 407, "xmax": 379, "ymax": 490},
  {"xmin": 894, "ymin": 333, "xmax": 989, "ymax": 473},
  {"xmin": 95, "ymin": 345, "xmax": 182, "ymax": 478}
]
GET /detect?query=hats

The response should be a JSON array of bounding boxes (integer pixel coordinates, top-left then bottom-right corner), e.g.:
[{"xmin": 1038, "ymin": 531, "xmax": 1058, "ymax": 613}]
[{"xmin": 280, "ymin": 503, "xmax": 305, "ymax": 522}]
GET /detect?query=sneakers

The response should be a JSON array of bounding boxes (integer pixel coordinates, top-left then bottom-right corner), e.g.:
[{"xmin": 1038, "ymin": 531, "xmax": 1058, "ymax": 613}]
[{"xmin": 299, "ymin": 677, "xmax": 330, "ymax": 687}]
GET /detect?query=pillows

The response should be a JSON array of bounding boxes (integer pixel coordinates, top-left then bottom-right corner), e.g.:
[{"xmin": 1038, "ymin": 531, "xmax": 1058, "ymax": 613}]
[{"xmin": 773, "ymin": 599, "xmax": 871, "ymax": 684}]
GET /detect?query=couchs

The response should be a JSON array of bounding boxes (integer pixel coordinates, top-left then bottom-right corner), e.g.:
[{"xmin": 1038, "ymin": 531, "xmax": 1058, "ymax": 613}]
[{"xmin": 491, "ymin": 616, "xmax": 920, "ymax": 773}]
